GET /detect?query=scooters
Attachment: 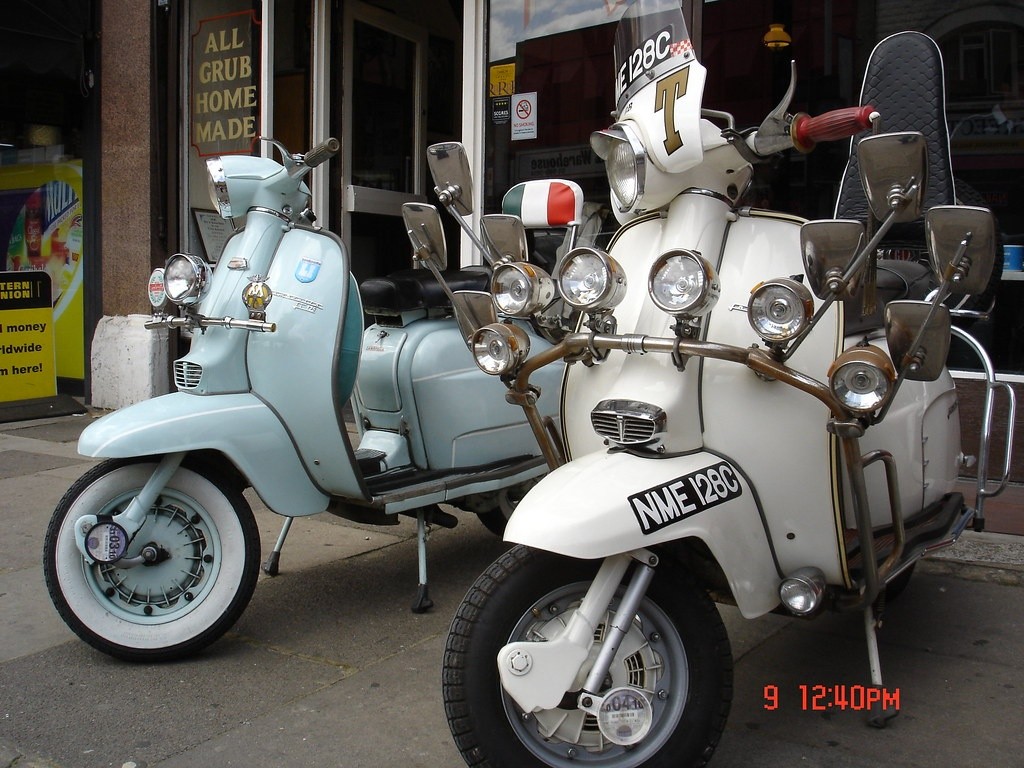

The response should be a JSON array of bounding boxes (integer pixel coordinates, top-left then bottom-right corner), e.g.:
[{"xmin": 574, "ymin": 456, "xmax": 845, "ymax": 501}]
[
  {"xmin": 36, "ymin": 135, "xmax": 594, "ymax": 665},
  {"xmin": 399, "ymin": 1, "xmax": 1024, "ymax": 768}
]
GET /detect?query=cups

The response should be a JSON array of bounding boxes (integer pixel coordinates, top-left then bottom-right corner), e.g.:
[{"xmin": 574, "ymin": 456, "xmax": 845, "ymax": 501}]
[{"xmin": 1003, "ymin": 245, "xmax": 1024, "ymax": 272}]
